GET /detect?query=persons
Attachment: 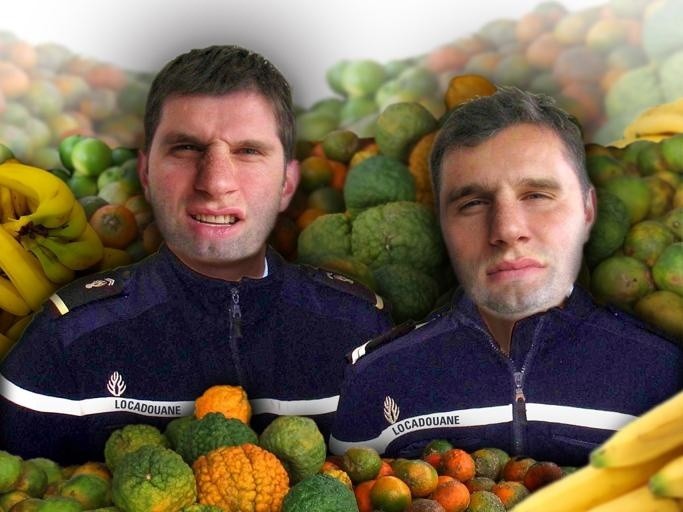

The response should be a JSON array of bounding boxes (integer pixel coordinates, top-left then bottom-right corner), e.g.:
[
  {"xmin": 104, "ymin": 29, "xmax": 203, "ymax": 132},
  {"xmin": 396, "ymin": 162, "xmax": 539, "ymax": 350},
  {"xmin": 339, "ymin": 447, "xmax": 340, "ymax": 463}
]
[
  {"xmin": 0, "ymin": 45, "xmax": 400, "ymax": 468},
  {"xmin": 326, "ymin": 85, "xmax": 681, "ymax": 470}
]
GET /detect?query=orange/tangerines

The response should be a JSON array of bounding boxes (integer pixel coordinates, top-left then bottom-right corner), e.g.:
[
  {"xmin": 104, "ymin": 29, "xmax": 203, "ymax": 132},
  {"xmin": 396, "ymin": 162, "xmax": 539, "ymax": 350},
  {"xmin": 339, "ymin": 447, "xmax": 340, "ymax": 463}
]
[{"xmin": 0, "ymin": 1, "xmax": 683, "ymax": 512}]
